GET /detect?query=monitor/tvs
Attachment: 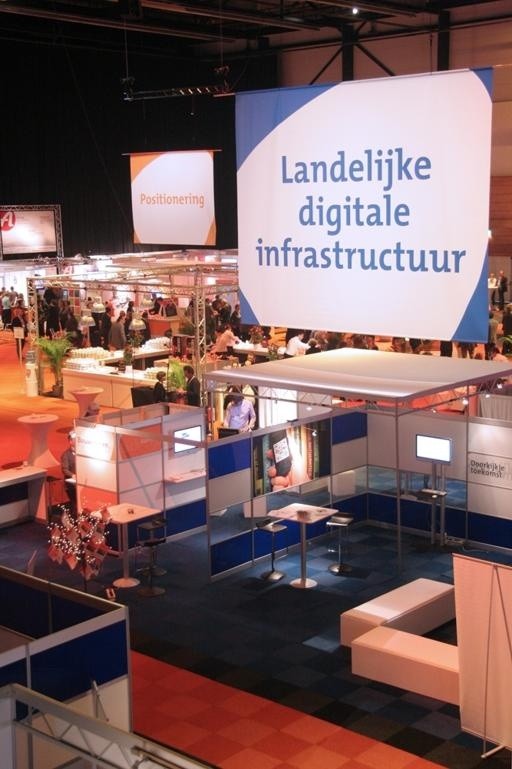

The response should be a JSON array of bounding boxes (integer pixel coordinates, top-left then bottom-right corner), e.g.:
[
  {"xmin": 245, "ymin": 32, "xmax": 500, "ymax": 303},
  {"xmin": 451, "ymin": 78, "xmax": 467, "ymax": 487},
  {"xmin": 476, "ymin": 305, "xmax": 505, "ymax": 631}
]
[
  {"xmin": 218, "ymin": 429, "xmax": 239, "ymax": 439},
  {"xmin": 415, "ymin": 433, "xmax": 453, "ymax": 465},
  {"xmin": 173, "ymin": 425, "xmax": 203, "ymax": 454}
]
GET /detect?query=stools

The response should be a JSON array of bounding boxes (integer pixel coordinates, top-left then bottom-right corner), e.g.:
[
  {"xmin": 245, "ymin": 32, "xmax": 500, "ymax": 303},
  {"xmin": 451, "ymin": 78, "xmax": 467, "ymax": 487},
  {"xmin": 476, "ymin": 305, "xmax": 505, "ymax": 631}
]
[
  {"xmin": 136, "ymin": 538, "xmax": 166, "ymax": 598},
  {"xmin": 136, "ymin": 518, "xmax": 168, "ymax": 577},
  {"xmin": 325, "ymin": 514, "xmax": 353, "ymax": 576},
  {"xmin": 259, "ymin": 522, "xmax": 287, "ymax": 581}
]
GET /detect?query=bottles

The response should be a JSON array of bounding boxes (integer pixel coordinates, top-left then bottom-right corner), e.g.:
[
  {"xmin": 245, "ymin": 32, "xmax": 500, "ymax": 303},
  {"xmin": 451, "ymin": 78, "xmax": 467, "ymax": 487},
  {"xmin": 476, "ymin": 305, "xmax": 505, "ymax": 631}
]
[
  {"xmin": 70, "ymin": 346, "xmax": 109, "ymax": 359},
  {"xmin": 144, "ymin": 367, "xmax": 168, "ymax": 381},
  {"xmin": 145, "ymin": 337, "xmax": 170, "ymax": 350},
  {"xmin": 64, "ymin": 358, "xmax": 101, "ymax": 371}
]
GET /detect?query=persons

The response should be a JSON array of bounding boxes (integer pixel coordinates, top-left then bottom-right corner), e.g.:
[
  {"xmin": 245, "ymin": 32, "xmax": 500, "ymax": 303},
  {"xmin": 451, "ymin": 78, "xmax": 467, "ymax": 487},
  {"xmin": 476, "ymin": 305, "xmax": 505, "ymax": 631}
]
[
  {"xmin": 42, "ymin": 286, "xmax": 194, "ymax": 349},
  {"xmin": 154, "ymin": 372, "xmax": 166, "ymax": 400},
  {"xmin": 184, "ymin": 366, "xmax": 200, "ymax": 406},
  {"xmin": 284, "ymin": 328, "xmax": 379, "ymax": 359},
  {"xmin": 206, "ymin": 295, "xmax": 271, "ymax": 364},
  {"xmin": 1, "ymin": 286, "xmax": 27, "ymax": 359},
  {"xmin": 476, "ymin": 271, "xmax": 512, "ymax": 362},
  {"xmin": 224, "ymin": 396, "xmax": 256, "ymax": 431},
  {"xmin": 266, "ymin": 437, "xmax": 313, "ymax": 493},
  {"xmin": 61, "ymin": 432, "xmax": 76, "ymax": 505},
  {"xmin": 388, "ymin": 337, "xmax": 477, "ymax": 358}
]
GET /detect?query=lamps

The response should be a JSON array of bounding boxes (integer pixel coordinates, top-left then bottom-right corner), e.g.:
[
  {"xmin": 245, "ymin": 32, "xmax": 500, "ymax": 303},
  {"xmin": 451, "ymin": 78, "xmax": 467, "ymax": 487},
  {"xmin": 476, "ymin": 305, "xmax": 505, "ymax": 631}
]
[
  {"xmin": 141, "ymin": 282, "xmax": 154, "ymax": 311},
  {"xmin": 79, "ymin": 286, "xmax": 107, "ymax": 327},
  {"xmin": 128, "ymin": 283, "xmax": 147, "ymax": 332}
]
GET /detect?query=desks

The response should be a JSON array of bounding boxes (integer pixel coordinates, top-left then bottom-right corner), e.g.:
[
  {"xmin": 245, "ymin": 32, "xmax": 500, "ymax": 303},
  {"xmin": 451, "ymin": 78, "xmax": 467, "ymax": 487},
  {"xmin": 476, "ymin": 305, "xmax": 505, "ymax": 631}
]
[
  {"xmin": 233, "ymin": 342, "xmax": 269, "ymax": 356},
  {"xmin": 148, "ymin": 316, "xmax": 180, "ymax": 336},
  {"xmin": 17, "ymin": 415, "xmax": 60, "ymax": 469},
  {"xmin": 266, "ymin": 502, "xmax": 339, "ymax": 591},
  {"xmin": 487, "ymin": 287, "xmax": 498, "ymax": 305},
  {"xmin": 99, "ymin": 347, "xmax": 170, "ymax": 370},
  {"xmin": 61, "ymin": 365, "xmax": 169, "ymax": 409},
  {"xmin": 68, "ymin": 387, "xmax": 104, "ymax": 417},
  {"xmin": 91, "ymin": 502, "xmax": 162, "ymax": 588}
]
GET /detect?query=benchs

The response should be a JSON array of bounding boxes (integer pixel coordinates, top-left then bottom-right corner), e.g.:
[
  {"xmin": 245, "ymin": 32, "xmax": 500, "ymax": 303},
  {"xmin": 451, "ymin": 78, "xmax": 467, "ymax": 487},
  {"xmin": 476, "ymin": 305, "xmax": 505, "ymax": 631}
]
[
  {"xmin": 351, "ymin": 626, "xmax": 459, "ymax": 706},
  {"xmin": 339, "ymin": 578, "xmax": 457, "ymax": 648}
]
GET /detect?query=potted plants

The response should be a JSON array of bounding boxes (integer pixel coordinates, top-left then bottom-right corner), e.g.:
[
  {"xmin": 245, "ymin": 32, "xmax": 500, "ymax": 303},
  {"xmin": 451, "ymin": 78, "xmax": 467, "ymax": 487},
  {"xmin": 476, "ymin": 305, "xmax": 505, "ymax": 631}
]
[{"xmin": 35, "ymin": 335, "xmax": 73, "ymax": 398}]
